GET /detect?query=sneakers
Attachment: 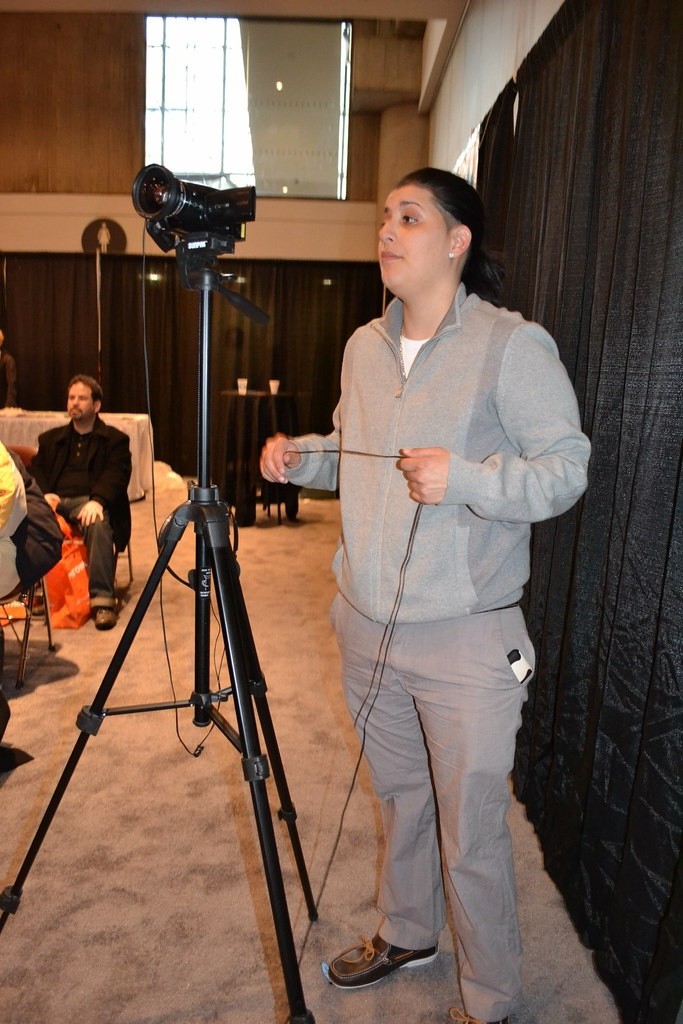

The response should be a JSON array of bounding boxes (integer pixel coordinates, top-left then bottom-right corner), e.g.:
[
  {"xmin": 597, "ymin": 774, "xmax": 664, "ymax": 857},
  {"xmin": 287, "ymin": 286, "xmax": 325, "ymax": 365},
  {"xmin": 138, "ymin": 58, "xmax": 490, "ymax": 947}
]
[
  {"xmin": 449, "ymin": 1006, "xmax": 509, "ymax": 1024},
  {"xmin": 95, "ymin": 607, "xmax": 115, "ymax": 627},
  {"xmin": 328, "ymin": 934, "xmax": 439, "ymax": 990},
  {"xmin": 31, "ymin": 605, "xmax": 46, "ymax": 614}
]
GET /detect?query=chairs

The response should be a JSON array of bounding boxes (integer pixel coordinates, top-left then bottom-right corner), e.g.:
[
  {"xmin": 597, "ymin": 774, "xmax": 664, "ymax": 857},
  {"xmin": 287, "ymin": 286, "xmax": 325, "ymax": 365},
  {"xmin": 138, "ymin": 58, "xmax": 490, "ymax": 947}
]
[{"xmin": 0, "ymin": 446, "xmax": 133, "ymax": 691}]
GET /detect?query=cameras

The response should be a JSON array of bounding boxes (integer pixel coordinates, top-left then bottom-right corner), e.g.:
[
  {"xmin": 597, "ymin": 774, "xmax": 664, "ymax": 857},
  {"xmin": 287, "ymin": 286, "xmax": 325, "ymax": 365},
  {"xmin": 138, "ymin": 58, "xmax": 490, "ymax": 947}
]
[{"xmin": 130, "ymin": 164, "xmax": 257, "ymax": 241}]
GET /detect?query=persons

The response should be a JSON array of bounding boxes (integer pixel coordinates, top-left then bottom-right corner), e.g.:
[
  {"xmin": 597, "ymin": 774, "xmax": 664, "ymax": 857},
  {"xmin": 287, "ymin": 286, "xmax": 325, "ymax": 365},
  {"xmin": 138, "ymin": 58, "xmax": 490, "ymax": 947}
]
[
  {"xmin": 0, "ymin": 439, "xmax": 64, "ymax": 750},
  {"xmin": 25, "ymin": 375, "xmax": 132, "ymax": 628},
  {"xmin": 258, "ymin": 168, "xmax": 592, "ymax": 1024}
]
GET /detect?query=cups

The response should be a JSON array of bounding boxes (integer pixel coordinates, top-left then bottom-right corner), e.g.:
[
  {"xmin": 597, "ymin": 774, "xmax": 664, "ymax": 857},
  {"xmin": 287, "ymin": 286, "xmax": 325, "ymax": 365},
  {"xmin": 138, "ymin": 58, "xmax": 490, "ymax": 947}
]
[
  {"xmin": 238, "ymin": 378, "xmax": 247, "ymax": 396},
  {"xmin": 269, "ymin": 380, "xmax": 279, "ymax": 394}
]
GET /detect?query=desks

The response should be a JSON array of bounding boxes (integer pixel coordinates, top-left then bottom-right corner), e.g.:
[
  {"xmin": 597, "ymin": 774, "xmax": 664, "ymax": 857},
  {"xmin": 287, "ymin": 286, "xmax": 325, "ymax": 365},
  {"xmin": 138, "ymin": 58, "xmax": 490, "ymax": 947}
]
[{"xmin": 0, "ymin": 410, "xmax": 155, "ymax": 504}]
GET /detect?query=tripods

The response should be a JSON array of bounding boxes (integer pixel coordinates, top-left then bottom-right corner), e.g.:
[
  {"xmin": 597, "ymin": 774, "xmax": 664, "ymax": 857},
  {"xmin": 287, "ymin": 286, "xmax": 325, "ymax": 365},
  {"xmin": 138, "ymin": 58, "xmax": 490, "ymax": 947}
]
[{"xmin": 0, "ymin": 237, "xmax": 317, "ymax": 1024}]
